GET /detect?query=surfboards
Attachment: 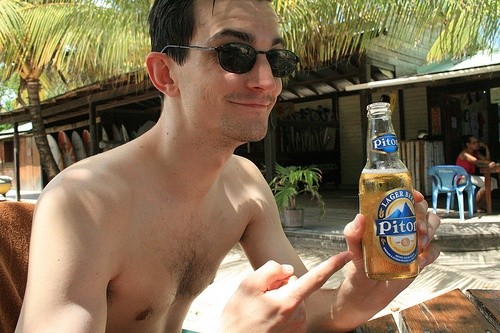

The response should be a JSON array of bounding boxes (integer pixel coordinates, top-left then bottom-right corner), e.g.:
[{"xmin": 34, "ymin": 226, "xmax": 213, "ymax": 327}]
[{"xmin": 46, "ymin": 123, "xmax": 130, "ymax": 172}]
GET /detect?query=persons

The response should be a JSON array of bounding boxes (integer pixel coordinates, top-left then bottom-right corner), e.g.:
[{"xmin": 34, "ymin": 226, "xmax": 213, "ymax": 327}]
[
  {"xmin": 455, "ymin": 133, "xmax": 498, "ymax": 213},
  {"xmin": 12, "ymin": 0, "xmax": 443, "ymax": 333}
]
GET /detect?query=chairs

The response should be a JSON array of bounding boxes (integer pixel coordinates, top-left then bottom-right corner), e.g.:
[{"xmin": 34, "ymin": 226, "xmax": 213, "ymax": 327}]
[{"xmin": 429, "ymin": 165, "xmax": 476, "ymax": 220}]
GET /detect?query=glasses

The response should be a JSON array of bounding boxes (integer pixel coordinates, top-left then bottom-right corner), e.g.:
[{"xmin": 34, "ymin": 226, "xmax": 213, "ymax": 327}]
[{"xmin": 161, "ymin": 41, "xmax": 300, "ymax": 78}]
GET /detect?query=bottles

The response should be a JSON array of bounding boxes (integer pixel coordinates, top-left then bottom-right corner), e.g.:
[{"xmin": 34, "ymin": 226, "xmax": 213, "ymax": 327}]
[{"xmin": 358, "ymin": 103, "xmax": 420, "ymax": 279}]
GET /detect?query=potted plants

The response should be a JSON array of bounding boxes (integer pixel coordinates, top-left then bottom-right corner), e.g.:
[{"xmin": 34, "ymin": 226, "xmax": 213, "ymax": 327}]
[{"xmin": 268, "ymin": 162, "xmax": 326, "ymax": 226}]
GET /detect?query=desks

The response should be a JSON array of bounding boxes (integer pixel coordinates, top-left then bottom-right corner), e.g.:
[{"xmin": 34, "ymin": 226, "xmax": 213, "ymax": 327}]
[
  {"xmin": 352, "ymin": 289, "xmax": 500, "ymax": 333},
  {"xmin": 477, "ymin": 163, "xmax": 500, "ymax": 215}
]
